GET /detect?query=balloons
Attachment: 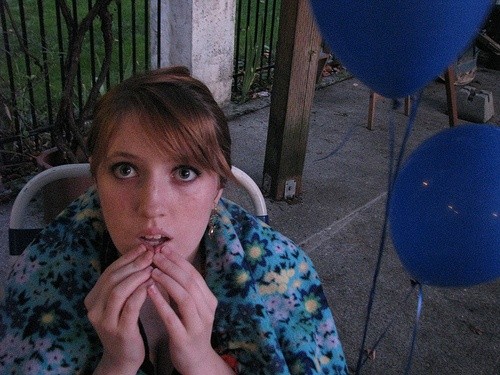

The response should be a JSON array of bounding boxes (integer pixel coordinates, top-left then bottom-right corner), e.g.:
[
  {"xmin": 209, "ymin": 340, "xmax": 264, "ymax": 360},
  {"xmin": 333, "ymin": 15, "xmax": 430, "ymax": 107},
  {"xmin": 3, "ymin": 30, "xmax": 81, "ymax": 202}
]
[
  {"xmin": 308, "ymin": 0, "xmax": 492, "ymax": 105},
  {"xmin": 388, "ymin": 125, "xmax": 500, "ymax": 288}
]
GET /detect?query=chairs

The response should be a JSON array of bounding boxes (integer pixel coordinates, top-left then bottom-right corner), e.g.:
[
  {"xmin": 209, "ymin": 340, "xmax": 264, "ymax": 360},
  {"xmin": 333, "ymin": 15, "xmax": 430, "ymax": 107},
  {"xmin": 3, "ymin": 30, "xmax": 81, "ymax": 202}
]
[{"xmin": 8, "ymin": 158, "xmax": 271, "ymax": 294}]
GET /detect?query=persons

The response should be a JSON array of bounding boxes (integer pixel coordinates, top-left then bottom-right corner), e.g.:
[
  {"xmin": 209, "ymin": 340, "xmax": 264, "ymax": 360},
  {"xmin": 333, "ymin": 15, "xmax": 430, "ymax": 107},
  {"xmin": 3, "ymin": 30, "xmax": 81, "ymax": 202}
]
[{"xmin": 0, "ymin": 66, "xmax": 351, "ymax": 375}]
